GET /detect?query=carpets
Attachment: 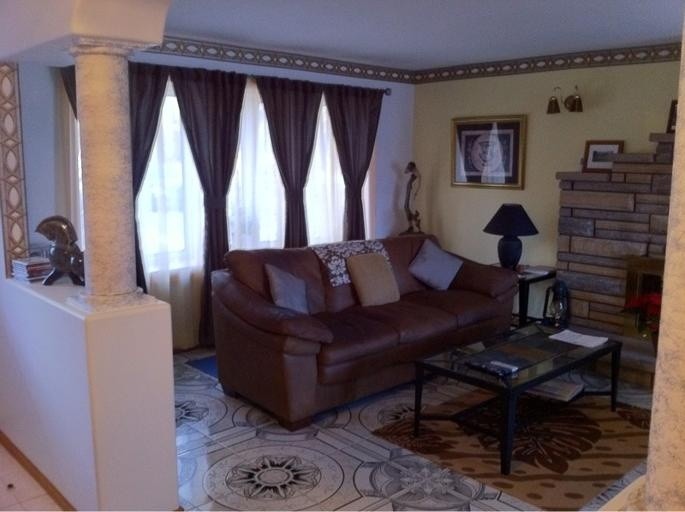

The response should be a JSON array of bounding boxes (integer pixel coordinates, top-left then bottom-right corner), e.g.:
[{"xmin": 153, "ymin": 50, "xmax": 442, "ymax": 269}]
[
  {"xmin": 185, "ymin": 351, "xmax": 429, "ymax": 423},
  {"xmin": 372, "ymin": 383, "xmax": 650, "ymax": 511}
]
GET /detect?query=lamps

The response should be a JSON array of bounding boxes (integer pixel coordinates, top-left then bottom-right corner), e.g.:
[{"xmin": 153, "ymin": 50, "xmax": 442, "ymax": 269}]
[
  {"xmin": 546, "ymin": 86, "xmax": 583, "ymax": 114},
  {"xmin": 485, "ymin": 202, "xmax": 539, "ymax": 271}
]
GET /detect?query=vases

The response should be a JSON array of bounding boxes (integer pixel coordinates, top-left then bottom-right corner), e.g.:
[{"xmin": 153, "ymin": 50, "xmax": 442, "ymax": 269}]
[{"xmin": 651, "ymin": 332, "xmax": 659, "ymax": 358}]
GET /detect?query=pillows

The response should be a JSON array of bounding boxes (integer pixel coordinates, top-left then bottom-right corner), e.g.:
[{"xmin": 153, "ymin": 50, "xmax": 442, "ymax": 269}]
[
  {"xmin": 264, "ymin": 263, "xmax": 310, "ymax": 315},
  {"xmin": 347, "ymin": 251, "xmax": 401, "ymax": 307},
  {"xmin": 408, "ymin": 238, "xmax": 464, "ymax": 291}
]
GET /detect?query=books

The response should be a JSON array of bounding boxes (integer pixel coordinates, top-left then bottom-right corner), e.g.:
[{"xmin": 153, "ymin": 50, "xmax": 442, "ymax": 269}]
[
  {"xmin": 10, "ymin": 256, "xmax": 53, "ymax": 285},
  {"xmin": 468, "ymin": 344, "xmax": 586, "ymax": 404}
]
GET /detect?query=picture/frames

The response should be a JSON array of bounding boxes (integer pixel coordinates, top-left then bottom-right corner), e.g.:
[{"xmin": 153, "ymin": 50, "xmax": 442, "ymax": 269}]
[
  {"xmin": 666, "ymin": 99, "xmax": 677, "ymax": 133},
  {"xmin": 450, "ymin": 114, "xmax": 528, "ymax": 190},
  {"xmin": 582, "ymin": 139, "xmax": 624, "ymax": 173}
]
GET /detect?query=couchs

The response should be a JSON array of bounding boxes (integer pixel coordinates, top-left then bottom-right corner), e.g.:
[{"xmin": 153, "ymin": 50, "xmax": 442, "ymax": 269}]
[{"xmin": 210, "ymin": 234, "xmax": 520, "ymax": 422}]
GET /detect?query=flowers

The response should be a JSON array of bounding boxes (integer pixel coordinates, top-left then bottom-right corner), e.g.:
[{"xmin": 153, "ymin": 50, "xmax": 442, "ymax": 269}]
[{"xmin": 624, "ymin": 292, "xmax": 661, "ymax": 331}]
